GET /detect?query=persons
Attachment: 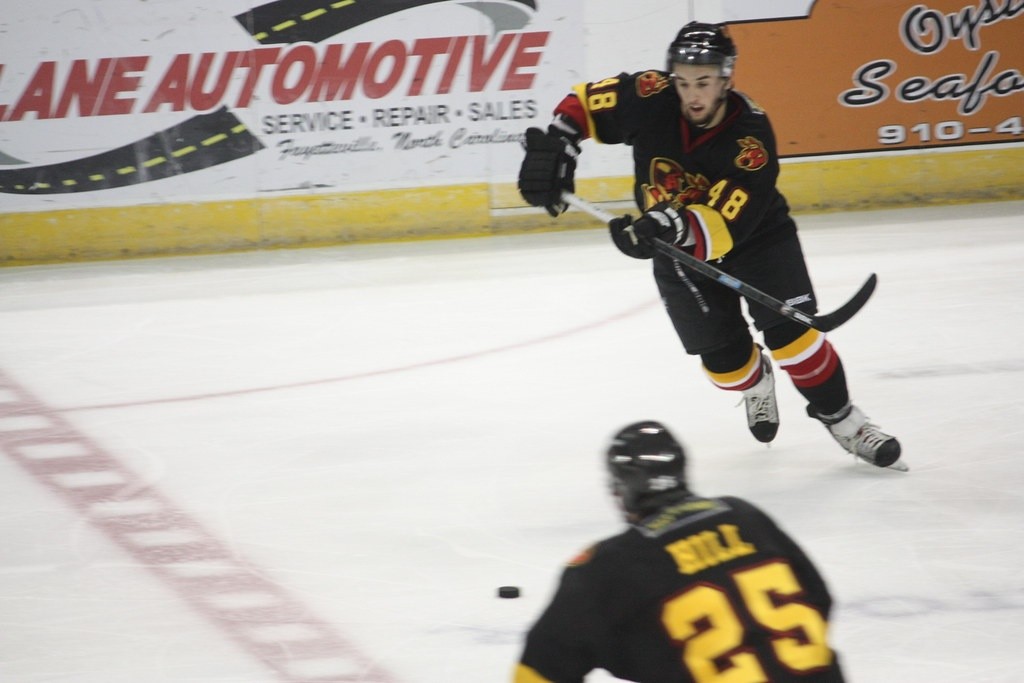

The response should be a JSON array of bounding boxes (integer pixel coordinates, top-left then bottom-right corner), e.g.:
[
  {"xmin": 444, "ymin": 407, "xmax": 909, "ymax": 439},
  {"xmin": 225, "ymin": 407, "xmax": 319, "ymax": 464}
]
[
  {"xmin": 517, "ymin": 22, "xmax": 903, "ymax": 470},
  {"xmin": 507, "ymin": 421, "xmax": 844, "ymax": 683}
]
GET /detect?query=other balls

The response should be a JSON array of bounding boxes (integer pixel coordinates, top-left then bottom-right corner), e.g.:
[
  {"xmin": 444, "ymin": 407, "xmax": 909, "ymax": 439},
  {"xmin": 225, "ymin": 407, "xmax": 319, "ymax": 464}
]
[{"xmin": 500, "ymin": 586, "xmax": 520, "ymax": 600}]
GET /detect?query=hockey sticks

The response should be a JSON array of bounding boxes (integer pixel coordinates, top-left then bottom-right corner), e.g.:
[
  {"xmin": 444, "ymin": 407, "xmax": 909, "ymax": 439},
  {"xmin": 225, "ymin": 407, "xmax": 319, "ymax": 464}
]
[{"xmin": 562, "ymin": 193, "xmax": 877, "ymax": 334}]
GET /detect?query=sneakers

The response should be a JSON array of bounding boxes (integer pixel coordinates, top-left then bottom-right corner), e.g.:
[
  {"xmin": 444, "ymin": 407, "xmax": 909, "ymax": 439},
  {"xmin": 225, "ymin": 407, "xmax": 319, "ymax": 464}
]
[
  {"xmin": 734, "ymin": 344, "xmax": 780, "ymax": 447},
  {"xmin": 807, "ymin": 397, "xmax": 907, "ymax": 472}
]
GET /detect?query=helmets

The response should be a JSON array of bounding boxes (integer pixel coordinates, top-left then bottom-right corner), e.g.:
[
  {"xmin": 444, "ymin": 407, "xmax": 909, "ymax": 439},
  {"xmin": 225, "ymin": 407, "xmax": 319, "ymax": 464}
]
[
  {"xmin": 669, "ymin": 20, "xmax": 739, "ymax": 80},
  {"xmin": 608, "ymin": 422, "xmax": 686, "ymax": 511}
]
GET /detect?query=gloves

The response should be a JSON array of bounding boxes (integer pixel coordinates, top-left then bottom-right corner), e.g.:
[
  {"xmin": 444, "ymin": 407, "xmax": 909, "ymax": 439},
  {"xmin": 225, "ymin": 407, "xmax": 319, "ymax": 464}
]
[
  {"xmin": 607, "ymin": 203, "xmax": 690, "ymax": 259},
  {"xmin": 520, "ymin": 123, "xmax": 582, "ymax": 216}
]
